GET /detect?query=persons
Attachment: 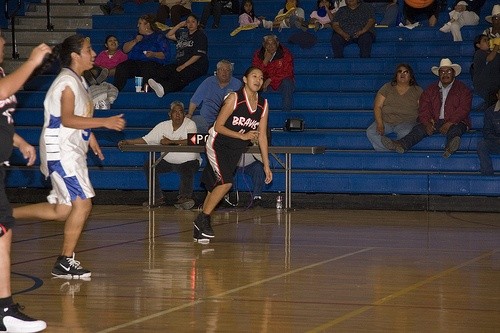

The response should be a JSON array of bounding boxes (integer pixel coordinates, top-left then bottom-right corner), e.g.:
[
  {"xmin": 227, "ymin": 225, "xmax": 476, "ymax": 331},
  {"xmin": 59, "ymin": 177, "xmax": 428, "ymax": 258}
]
[
  {"xmin": 439, "ymin": 0, "xmax": 485, "ymax": 43},
  {"xmin": 309, "ymin": 0, "xmax": 337, "ymax": 28},
  {"xmin": 0, "ymin": 35, "xmax": 53, "ymax": 332},
  {"xmin": 272, "ymin": 0, "xmax": 305, "ymax": 30},
  {"xmin": 250, "ymin": 32, "xmax": 297, "ymax": 112},
  {"xmin": 187, "ymin": 59, "xmax": 245, "ymax": 144},
  {"xmin": 118, "ymin": 98, "xmax": 204, "ymax": 211},
  {"xmin": 380, "ymin": 57, "xmax": 474, "ymax": 159},
  {"xmin": 388, "ymin": 0, "xmax": 444, "ymax": 29},
  {"xmin": 469, "ymin": 3, "xmax": 500, "ymax": 176},
  {"xmin": 365, "ymin": 63, "xmax": 424, "ymax": 153},
  {"xmin": 220, "ymin": 135, "xmax": 268, "ymax": 209},
  {"xmin": 148, "ymin": 0, "xmax": 193, "ymax": 31},
  {"xmin": 193, "ymin": 65, "xmax": 273, "ymax": 243},
  {"xmin": 329, "ymin": 0, "xmax": 377, "ymax": 59},
  {"xmin": 198, "ymin": 0, "xmax": 241, "ymax": 31},
  {"xmin": 236, "ymin": 0, "xmax": 266, "ymax": 31},
  {"xmin": 147, "ymin": 14, "xmax": 210, "ymax": 98},
  {"xmin": 81, "ymin": 66, "xmax": 120, "ymax": 110},
  {"xmin": 82, "ymin": 34, "xmax": 129, "ymax": 86},
  {"xmin": 9, "ymin": 32, "xmax": 127, "ymax": 280},
  {"xmin": 113, "ymin": 14, "xmax": 171, "ymax": 92}
]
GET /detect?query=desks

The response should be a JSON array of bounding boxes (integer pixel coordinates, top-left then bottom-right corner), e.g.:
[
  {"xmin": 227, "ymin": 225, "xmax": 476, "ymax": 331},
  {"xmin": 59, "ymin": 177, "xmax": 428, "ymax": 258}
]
[{"xmin": 119, "ymin": 144, "xmax": 326, "ymax": 210}]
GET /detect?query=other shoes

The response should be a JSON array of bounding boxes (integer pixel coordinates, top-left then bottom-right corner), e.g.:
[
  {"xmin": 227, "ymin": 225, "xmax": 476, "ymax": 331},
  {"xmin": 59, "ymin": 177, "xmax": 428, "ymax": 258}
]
[
  {"xmin": 442, "ymin": 136, "xmax": 461, "ymax": 158},
  {"xmin": 238, "ymin": 5, "xmax": 441, "ymax": 36},
  {"xmin": 196, "ymin": 24, "xmax": 206, "ymax": 30},
  {"xmin": 210, "ymin": 24, "xmax": 219, "ymax": 28},
  {"xmin": 84, "ymin": 67, "xmax": 109, "ymax": 86},
  {"xmin": 148, "ymin": 79, "xmax": 164, "ymax": 98},
  {"xmin": 380, "ymin": 135, "xmax": 409, "ymax": 154}
]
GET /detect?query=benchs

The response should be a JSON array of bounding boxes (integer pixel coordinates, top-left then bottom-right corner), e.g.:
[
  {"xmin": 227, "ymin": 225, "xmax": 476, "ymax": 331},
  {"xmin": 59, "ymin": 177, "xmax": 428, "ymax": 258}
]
[{"xmin": 0, "ymin": 1, "xmax": 500, "ymax": 195}]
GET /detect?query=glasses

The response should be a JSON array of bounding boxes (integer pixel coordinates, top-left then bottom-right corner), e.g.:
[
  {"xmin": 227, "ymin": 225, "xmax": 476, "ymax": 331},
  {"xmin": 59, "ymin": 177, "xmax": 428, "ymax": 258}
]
[
  {"xmin": 492, "ymin": 16, "xmax": 500, "ymax": 20},
  {"xmin": 397, "ymin": 70, "xmax": 410, "ymax": 74},
  {"xmin": 439, "ymin": 70, "xmax": 453, "ymax": 74}
]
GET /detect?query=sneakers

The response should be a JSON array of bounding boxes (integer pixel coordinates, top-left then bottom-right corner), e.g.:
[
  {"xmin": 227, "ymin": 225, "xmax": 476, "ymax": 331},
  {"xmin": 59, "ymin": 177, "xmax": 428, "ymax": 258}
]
[
  {"xmin": 0, "ymin": 303, "xmax": 47, "ymax": 333},
  {"xmin": 50, "ymin": 256, "xmax": 92, "ymax": 279},
  {"xmin": 170, "ymin": 194, "xmax": 195, "ymax": 210},
  {"xmin": 252, "ymin": 197, "xmax": 264, "ymax": 208},
  {"xmin": 143, "ymin": 195, "xmax": 168, "ymax": 208},
  {"xmin": 192, "ymin": 211, "xmax": 216, "ymax": 242}
]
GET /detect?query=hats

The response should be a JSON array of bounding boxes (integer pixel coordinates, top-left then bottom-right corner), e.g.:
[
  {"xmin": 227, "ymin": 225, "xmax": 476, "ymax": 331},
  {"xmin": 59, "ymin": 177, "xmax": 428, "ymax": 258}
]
[
  {"xmin": 431, "ymin": 58, "xmax": 462, "ymax": 77},
  {"xmin": 485, "ymin": 4, "xmax": 500, "ymax": 23}
]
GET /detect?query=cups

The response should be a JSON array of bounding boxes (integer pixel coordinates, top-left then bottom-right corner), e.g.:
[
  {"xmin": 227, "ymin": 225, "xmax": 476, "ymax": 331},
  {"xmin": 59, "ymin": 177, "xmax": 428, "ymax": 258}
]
[{"xmin": 134, "ymin": 75, "xmax": 144, "ymax": 93}]
